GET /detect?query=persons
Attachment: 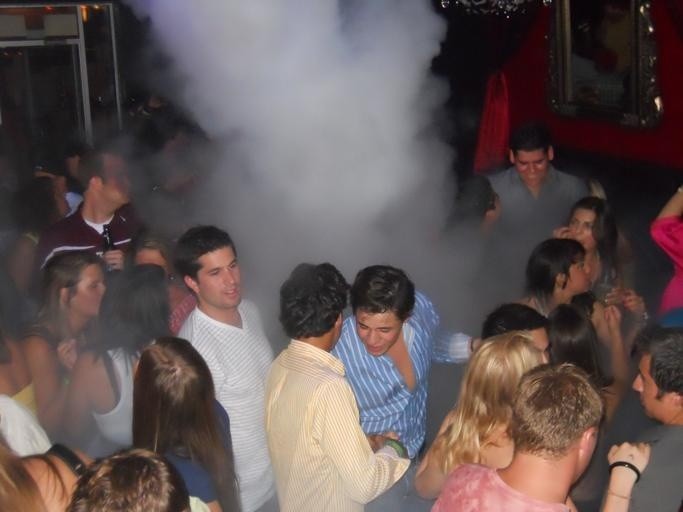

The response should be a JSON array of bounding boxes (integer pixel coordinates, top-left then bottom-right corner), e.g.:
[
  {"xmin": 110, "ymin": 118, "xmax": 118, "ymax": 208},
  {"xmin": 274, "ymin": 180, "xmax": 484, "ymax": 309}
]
[
  {"xmin": 1, "ymin": 94, "xmax": 683, "ymax": 512},
  {"xmin": 570, "ymin": 0, "xmax": 634, "ymax": 112}
]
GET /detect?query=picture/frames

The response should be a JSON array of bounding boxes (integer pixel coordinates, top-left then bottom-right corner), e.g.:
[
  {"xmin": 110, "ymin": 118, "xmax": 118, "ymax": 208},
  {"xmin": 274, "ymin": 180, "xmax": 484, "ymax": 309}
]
[{"xmin": 544, "ymin": 0, "xmax": 663, "ymax": 131}]
